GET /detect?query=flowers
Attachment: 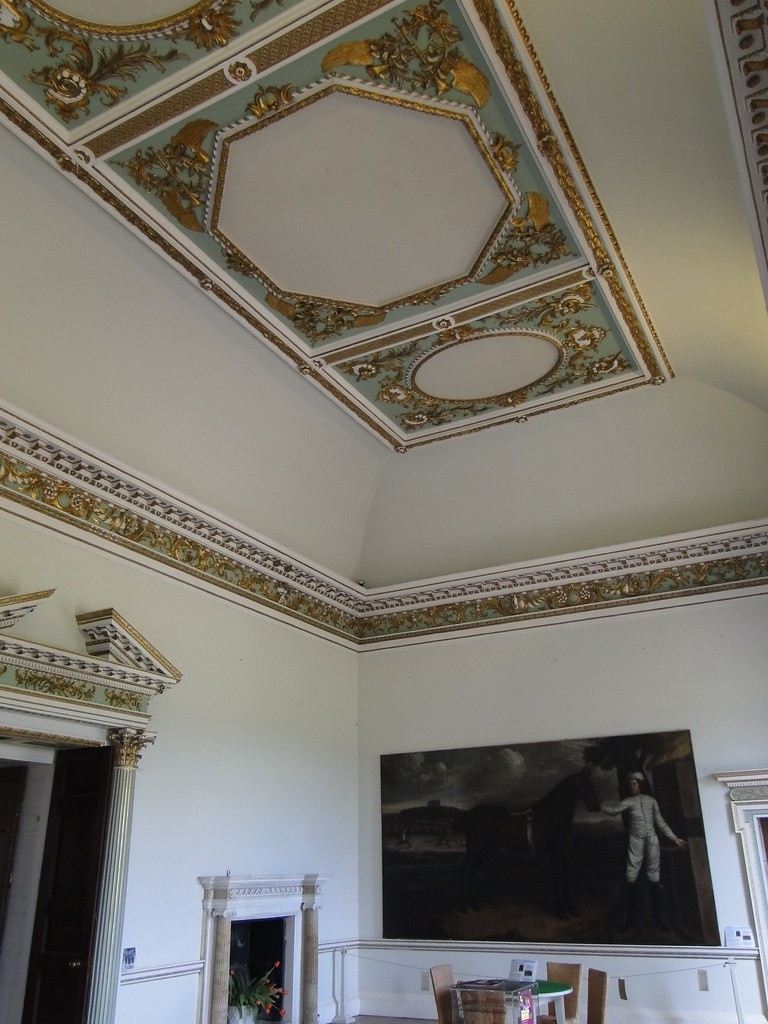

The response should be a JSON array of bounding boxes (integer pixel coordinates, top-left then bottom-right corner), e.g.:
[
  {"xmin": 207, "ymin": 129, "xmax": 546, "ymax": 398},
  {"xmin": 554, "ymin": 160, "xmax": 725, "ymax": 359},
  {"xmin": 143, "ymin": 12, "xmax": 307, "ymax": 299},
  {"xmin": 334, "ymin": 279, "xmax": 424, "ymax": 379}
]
[{"xmin": 228, "ymin": 960, "xmax": 288, "ymax": 1024}]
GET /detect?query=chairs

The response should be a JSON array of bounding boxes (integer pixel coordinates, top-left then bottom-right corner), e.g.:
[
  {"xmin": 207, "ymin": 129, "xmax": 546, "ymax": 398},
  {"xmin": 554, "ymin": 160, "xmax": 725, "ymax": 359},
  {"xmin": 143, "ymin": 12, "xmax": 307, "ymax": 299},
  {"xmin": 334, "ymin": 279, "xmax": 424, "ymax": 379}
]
[{"xmin": 430, "ymin": 961, "xmax": 608, "ymax": 1024}]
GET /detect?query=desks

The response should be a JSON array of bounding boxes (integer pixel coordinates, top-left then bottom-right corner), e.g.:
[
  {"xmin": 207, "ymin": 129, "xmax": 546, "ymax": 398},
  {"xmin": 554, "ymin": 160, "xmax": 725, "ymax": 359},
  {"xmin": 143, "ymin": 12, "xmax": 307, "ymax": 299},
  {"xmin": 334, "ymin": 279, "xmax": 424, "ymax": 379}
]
[{"xmin": 448, "ymin": 980, "xmax": 573, "ymax": 1024}]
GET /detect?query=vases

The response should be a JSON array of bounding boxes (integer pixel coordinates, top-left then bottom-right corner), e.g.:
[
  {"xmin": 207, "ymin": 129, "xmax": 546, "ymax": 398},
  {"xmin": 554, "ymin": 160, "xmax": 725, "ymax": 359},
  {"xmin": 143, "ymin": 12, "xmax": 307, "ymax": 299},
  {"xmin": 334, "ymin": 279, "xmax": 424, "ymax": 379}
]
[{"xmin": 227, "ymin": 1005, "xmax": 255, "ymax": 1024}]
